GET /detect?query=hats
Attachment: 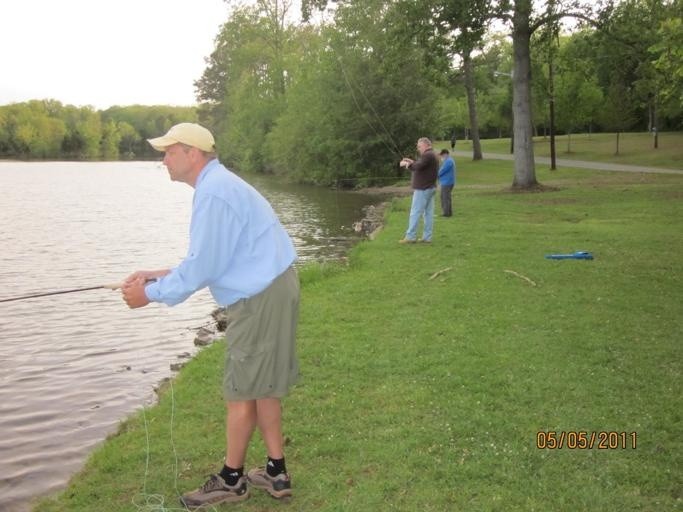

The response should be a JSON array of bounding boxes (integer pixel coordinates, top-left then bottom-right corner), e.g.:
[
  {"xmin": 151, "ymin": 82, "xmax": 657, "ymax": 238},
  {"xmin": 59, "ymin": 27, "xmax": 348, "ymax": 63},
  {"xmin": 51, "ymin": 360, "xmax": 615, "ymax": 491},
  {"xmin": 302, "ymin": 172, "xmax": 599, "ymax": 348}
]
[{"xmin": 144, "ymin": 121, "xmax": 218, "ymax": 155}]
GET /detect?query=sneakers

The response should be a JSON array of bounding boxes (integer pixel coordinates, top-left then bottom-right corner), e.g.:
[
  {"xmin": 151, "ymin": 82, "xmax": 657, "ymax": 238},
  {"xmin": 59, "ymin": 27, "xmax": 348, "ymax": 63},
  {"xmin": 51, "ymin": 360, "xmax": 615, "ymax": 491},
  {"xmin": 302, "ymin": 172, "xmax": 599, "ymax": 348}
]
[
  {"xmin": 397, "ymin": 238, "xmax": 431, "ymax": 245},
  {"xmin": 246, "ymin": 463, "xmax": 295, "ymax": 501},
  {"xmin": 176, "ymin": 471, "xmax": 251, "ymax": 510}
]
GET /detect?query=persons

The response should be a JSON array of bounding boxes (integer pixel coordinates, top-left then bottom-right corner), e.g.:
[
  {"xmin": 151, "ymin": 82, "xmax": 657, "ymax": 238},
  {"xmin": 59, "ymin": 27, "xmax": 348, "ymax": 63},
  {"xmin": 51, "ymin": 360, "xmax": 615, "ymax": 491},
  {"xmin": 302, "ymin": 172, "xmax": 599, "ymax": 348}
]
[
  {"xmin": 396, "ymin": 136, "xmax": 439, "ymax": 244},
  {"xmin": 119, "ymin": 122, "xmax": 300, "ymax": 510},
  {"xmin": 436, "ymin": 149, "xmax": 456, "ymax": 216}
]
